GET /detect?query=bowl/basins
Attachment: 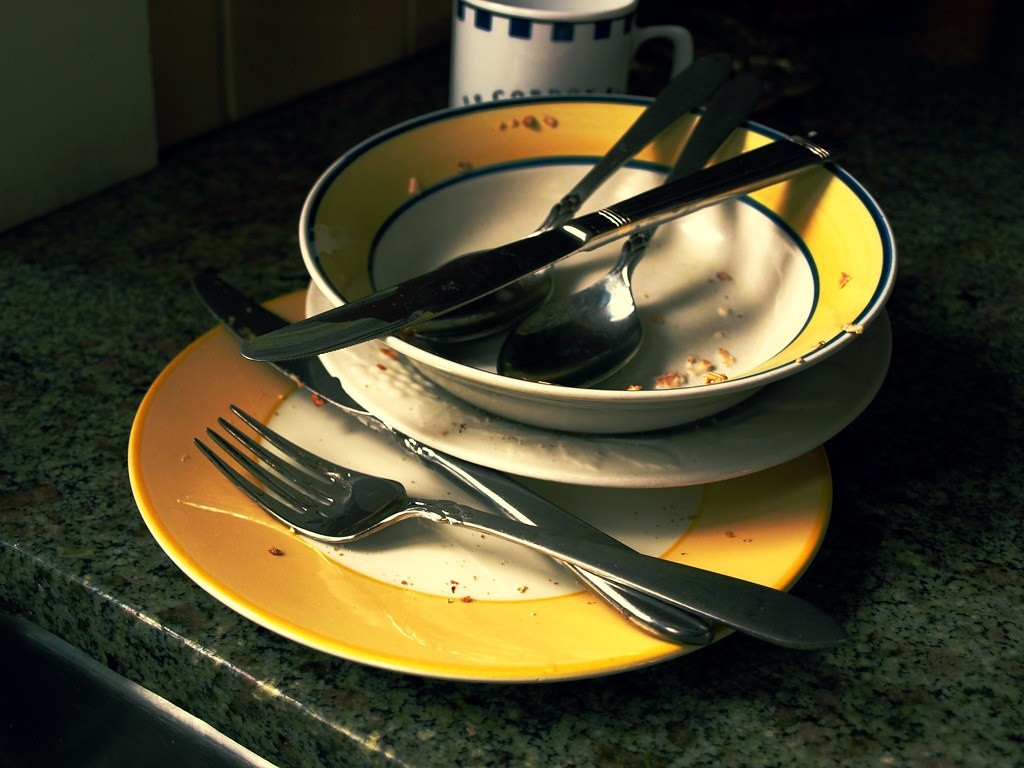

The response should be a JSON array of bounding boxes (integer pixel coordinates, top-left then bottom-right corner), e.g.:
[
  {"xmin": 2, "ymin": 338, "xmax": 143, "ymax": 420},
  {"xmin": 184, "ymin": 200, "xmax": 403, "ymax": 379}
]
[{"xmin": 295, "ymin": 91, "xmax": 896, "ymax": 433}]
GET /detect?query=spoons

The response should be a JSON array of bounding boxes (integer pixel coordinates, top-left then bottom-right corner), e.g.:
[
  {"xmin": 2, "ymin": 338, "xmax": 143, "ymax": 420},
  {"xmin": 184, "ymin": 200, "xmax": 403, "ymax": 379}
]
[
  {"xmin": 409, "ymin": 56, "xmax": 727, "ymax": 345},
  {"xmin": 497, "ymin": 68, "xmax": 787, "ymax": 383}
]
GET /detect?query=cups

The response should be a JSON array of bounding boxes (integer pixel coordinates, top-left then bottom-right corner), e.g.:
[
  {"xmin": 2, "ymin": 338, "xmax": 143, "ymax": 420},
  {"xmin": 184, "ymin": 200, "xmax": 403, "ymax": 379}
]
[{"xmin": 448, "ymin": 0, "xmax": 694, "ymax": 108}]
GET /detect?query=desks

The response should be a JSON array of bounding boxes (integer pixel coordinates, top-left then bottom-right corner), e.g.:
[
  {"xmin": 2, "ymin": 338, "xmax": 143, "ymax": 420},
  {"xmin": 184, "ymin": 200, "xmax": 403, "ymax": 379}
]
[{"xmin": 1, "ymin": 0, "xmax": 1024, "ymax": 768}]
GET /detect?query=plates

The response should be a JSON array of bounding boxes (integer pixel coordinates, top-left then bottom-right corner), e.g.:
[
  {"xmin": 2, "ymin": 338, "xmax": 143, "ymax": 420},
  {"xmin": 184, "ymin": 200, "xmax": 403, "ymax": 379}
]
[
  {"xmin": 125, "ymin": 279, "xmax": 837, "ymax": 680},
  {"xmin": 301, "ymin": 280, "xmax": 894, "ymax": 486}
]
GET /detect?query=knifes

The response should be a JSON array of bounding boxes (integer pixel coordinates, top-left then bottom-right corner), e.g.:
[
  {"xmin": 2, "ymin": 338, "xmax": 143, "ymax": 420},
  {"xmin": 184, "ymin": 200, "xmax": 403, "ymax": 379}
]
[
  {"xmin": 236, "ymin": 126, "xmax": 854, "ymax": 366},
  {"xmin": 190, "ymin": 268, "xmax": 716, "ymax": 647}
]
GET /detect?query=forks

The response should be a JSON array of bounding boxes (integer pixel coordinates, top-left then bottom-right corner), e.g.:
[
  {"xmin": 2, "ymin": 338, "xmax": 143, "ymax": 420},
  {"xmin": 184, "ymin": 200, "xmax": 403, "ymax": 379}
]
[{"xmin": 193, "ymin": 396, "xmax": 849, "ymax": 654}]
